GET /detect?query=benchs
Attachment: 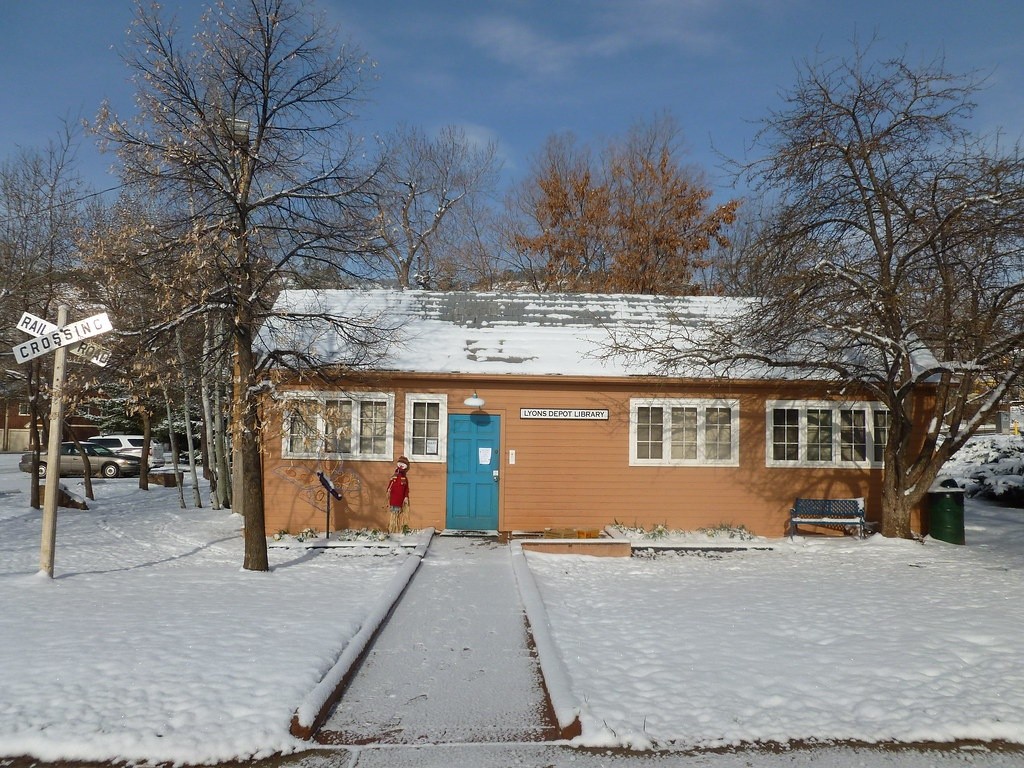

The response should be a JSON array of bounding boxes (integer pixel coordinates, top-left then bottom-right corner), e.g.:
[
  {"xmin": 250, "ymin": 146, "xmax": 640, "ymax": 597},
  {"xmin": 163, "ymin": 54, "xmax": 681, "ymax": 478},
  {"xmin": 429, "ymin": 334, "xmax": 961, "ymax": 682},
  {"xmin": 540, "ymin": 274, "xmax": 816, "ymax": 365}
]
[{"xmin": 788, "ymin": 496, "xmax": 865, "ymax": 541}]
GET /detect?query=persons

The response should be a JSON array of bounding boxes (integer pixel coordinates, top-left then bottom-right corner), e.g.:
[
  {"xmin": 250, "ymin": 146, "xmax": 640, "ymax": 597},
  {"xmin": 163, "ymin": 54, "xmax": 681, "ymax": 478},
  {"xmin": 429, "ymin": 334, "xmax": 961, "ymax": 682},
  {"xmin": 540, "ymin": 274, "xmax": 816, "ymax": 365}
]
[{"xmin": 382, "ymin": 456, "xmax": 411, "ymax": 533}]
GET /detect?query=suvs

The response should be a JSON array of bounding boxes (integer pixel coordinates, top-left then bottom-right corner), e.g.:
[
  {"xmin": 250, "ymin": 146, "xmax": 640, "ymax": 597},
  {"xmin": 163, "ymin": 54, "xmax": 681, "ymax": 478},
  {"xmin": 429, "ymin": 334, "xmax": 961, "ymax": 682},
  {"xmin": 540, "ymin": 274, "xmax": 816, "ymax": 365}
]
[{"xmin": 69, "ymin": 435, "xmax": 165, "ymax": 472}]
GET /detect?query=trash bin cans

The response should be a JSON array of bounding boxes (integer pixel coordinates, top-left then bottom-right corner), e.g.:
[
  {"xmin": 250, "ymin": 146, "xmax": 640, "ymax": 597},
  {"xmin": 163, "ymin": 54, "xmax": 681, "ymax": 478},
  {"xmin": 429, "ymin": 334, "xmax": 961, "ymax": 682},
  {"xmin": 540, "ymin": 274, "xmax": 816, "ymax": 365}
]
[{"xmin": 927, "ymin": 474, "xmax": 967, "ymax": 547}]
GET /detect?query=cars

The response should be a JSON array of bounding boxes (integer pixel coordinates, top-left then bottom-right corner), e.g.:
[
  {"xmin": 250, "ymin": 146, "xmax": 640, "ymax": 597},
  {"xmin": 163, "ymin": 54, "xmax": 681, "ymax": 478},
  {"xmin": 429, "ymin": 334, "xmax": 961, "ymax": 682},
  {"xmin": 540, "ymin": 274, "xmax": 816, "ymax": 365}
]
[{"xmin": 18, "ymin": 440, "xmax": 150, "ymax": 479}]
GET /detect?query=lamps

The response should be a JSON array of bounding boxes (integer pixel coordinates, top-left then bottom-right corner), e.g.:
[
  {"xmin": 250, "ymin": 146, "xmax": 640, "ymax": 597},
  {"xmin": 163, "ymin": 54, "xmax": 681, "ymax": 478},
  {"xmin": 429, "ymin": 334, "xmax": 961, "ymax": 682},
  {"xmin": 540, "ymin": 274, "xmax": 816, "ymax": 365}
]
[{"xmin": 463, "ymin": 384, "xmax": 485, "ymax": 409}]
